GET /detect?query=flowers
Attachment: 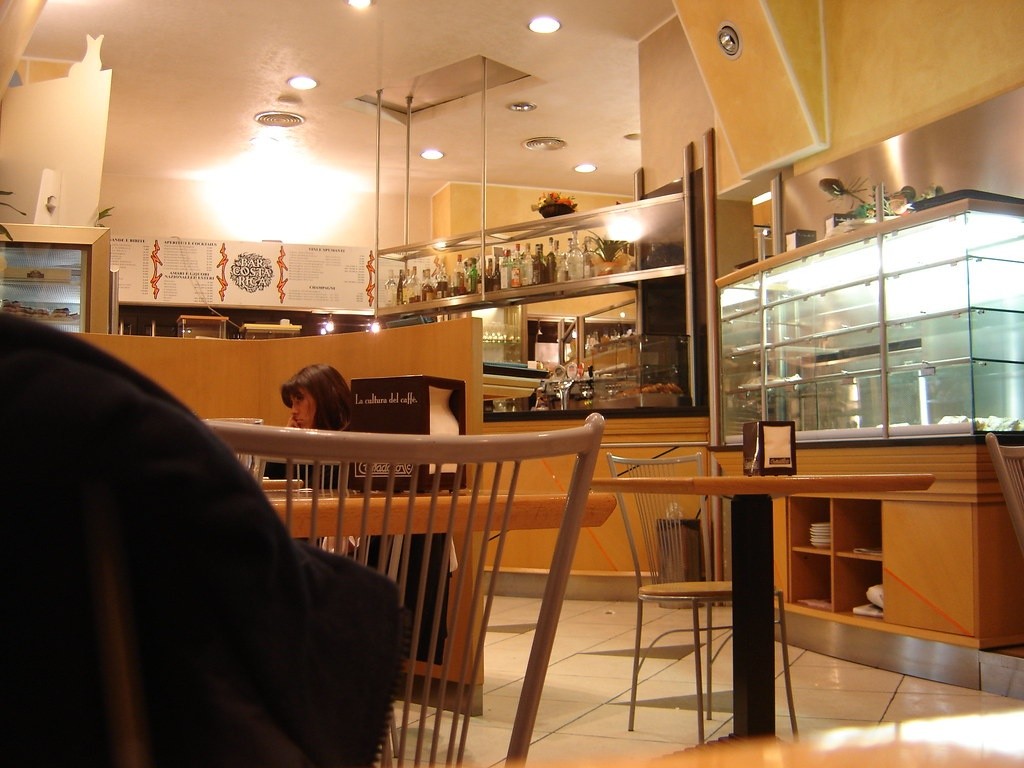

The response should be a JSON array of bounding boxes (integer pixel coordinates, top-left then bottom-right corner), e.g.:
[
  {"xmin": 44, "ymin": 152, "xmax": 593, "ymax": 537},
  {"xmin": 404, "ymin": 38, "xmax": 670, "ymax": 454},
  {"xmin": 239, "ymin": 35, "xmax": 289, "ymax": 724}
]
[{"xmin": 532, "ymin": 191, "xmax": 577, "ymax": 211}]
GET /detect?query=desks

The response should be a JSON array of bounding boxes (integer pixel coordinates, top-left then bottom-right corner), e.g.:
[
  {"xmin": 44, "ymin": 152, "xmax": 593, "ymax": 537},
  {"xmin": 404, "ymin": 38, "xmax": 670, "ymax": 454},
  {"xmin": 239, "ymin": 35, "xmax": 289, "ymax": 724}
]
[
  {"xmin": 588, "ymin": 474, "xmax": 934, "ymax": 752},
  {"xmin": 268, "ymin": 495, "xmax": 617, "ymax": 540}
]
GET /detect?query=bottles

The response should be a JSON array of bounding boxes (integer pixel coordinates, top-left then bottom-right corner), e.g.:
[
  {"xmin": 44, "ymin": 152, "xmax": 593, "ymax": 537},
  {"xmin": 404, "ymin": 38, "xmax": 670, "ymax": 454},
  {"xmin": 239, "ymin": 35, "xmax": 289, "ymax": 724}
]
[{"xmin": 384, "ymin": 237, "xmax": 591, "ymax": 305}]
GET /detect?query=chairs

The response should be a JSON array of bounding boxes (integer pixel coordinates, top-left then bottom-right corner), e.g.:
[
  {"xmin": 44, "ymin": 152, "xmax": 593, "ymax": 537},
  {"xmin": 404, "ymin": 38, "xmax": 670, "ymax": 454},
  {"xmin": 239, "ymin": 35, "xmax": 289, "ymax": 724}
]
[
  {"xmin": 96, "ymin": 413, "xmax": 607, "ymax": 768},
  {"xmin": 602, "ymin": 451, "xmax": 798, "ymax": 748},
  {"xmin": 985, "ymin": 432, "xmax": 1024, "ymax": 554}
]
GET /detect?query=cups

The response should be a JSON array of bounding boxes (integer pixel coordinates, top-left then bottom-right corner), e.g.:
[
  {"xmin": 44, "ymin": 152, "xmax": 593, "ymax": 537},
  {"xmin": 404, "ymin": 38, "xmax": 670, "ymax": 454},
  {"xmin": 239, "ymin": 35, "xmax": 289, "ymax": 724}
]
[{"xmin": 203, "ymin": 418, "xmax": 262, "ymax": 486}]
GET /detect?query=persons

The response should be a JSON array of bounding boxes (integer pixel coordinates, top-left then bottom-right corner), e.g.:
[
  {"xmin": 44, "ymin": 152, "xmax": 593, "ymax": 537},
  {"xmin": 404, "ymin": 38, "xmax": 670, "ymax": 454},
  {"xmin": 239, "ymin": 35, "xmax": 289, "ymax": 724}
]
[
  {"xmin": 259, "ymin": 364, "xmax": 375, "ymax": 553},
  {"xmin": 0, "ymin": 311, "xmax": 397, "ymax": 768}
]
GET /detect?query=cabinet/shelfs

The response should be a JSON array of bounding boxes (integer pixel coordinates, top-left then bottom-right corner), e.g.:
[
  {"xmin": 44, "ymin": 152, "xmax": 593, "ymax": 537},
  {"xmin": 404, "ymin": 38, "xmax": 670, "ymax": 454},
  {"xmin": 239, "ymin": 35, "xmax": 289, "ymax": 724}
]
[
  {"xmin": 373, "ymin": 141, "xmax": 698, "ymax": 414},
  {"xmin": 715, "ymin": 192, "xmax": 1024, "ymax": 639}
]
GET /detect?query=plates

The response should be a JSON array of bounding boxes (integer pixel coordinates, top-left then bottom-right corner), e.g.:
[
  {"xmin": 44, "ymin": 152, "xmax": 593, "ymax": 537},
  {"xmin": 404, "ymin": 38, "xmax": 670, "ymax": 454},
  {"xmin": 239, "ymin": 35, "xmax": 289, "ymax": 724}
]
[{"xmin": 809, "ymin": 522, "xmax": 830, "ymax": 547}]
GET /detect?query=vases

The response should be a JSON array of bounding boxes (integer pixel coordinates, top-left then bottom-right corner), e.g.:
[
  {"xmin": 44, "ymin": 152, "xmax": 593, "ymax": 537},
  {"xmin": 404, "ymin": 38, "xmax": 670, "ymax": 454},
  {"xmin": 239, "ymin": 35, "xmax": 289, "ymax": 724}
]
[{"xmin": 539, "ymin": 204, "xmax": 574, "ymax": 220}]
